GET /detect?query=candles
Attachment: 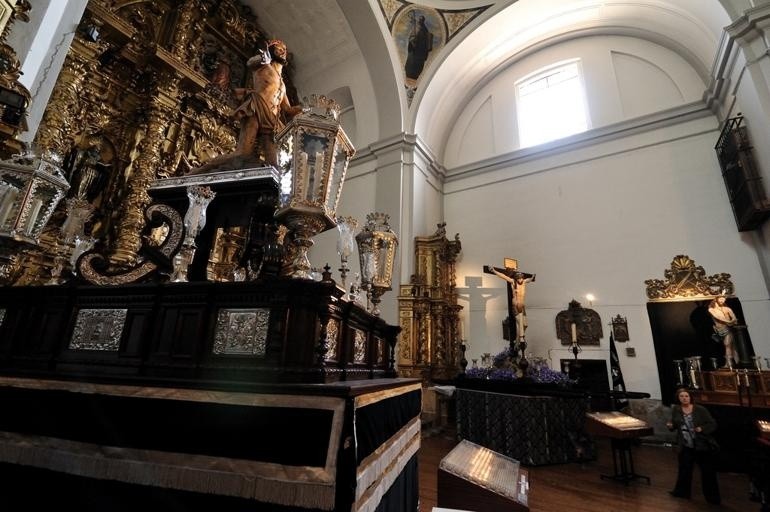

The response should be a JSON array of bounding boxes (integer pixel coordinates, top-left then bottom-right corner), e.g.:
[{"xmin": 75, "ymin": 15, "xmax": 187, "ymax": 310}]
[{"xmin": 571, "ymin": 323, "xmax": 577, "ymax": 347}]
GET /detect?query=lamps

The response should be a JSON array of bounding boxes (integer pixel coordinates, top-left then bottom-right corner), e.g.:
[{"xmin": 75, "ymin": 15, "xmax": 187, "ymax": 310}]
[
  {"xmin": 0, "ymin": 151, "xmax": 73, "ymax": 285},
  {"xmin": 272, "ymin": 95, "xmax": 358, "ymax": 276},
  {"xmin": 354, "ymin": 212, "xmax": 399, "ymax": 316}
]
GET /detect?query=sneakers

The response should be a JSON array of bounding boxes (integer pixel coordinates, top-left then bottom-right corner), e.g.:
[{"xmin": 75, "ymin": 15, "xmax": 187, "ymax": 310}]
[{"xmin": 667, "ymin": 490, "xmax": 692, "ymax": 499}]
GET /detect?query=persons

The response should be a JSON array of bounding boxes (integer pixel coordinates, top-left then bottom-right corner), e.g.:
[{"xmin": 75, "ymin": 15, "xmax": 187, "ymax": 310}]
[
  {"xmin": 487, "ymin": 265, "xmax": 535, "ymax": 349},
  {"xmin": 406, "ymin": 14, "xmax": 433, "ymax": 81},
  {"xmin": 664, "ymin": 386, "xmax": 724, "ymax": 507},
  {"xmin": 180, "ymin": 35, "xmax": 305, "ymax": 177},
  {"xmin": 708, "ymin": 292, "xmax": 742, "ymax": 369}
]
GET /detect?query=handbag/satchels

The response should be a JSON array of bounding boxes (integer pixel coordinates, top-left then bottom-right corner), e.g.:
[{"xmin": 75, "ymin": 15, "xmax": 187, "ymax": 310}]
[{"xmin": 693, "ymin": 438, "xmax": 726, "ymax": 471}]
